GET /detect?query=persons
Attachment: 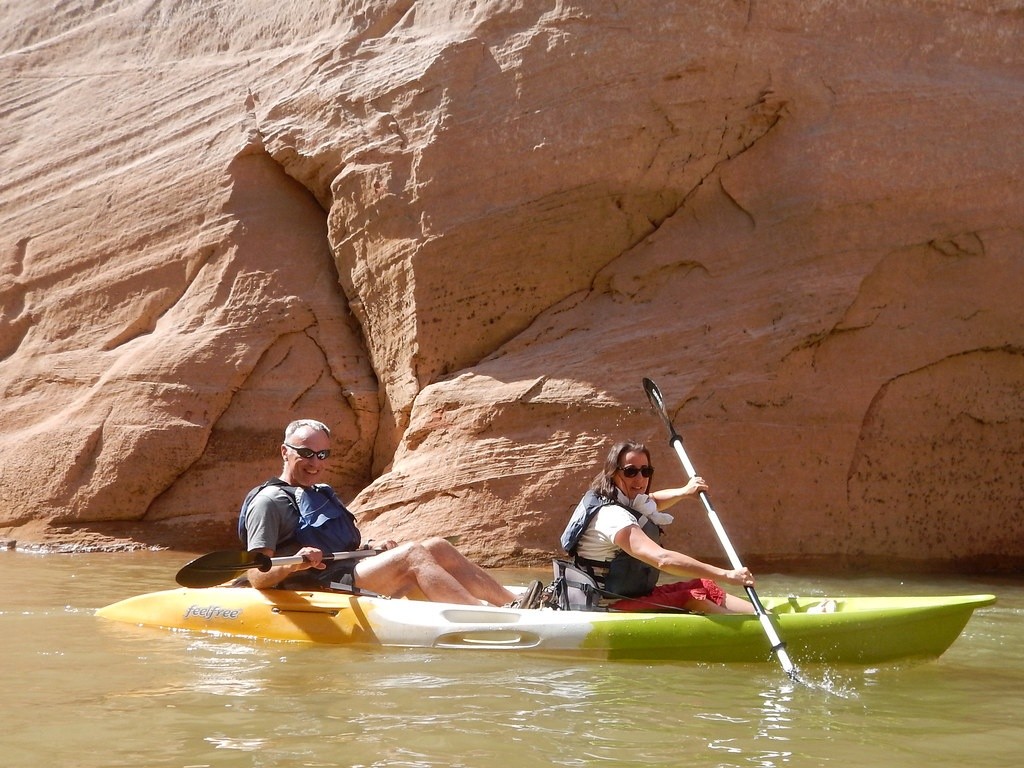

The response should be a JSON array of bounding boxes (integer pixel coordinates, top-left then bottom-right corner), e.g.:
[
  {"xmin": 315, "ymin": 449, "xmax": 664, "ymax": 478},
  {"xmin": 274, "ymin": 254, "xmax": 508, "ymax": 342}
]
[
  {"xmin": 559, "ymin": 440, "xmax": 771, "ymax": 618},
  {"xmin": 238, "ymin": 419, "xmax": 543, "ymax": 610}
]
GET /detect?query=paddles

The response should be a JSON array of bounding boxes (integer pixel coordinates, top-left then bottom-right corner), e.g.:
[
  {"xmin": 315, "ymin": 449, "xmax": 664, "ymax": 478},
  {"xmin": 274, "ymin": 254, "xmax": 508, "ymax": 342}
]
[
  {"xmin": 174, "ymin": 549, "xmax": 385, "ymax": 589},
  {"xmin": 641, "ymin": 376, "xmax": 796, "ymax": 674}
]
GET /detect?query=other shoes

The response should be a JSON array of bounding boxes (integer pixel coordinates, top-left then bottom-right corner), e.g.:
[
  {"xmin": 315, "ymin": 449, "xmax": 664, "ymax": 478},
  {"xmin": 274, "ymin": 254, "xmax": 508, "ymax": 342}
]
[{"xmin": 807, "ymin": 600, "xmax": 837, "ymax": 614}]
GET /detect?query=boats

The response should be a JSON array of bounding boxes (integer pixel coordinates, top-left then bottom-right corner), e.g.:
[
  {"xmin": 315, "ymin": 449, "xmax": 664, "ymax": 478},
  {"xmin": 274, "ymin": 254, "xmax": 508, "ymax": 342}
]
[{"xmin": 92, "ymin": 581, "xmax": 996, "ymax": 669}]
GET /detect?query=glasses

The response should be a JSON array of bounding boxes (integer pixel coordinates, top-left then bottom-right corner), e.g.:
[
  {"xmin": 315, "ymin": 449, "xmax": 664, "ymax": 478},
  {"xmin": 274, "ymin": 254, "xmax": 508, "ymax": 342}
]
[
  {"xmin": 286, "ymin": 443, "xmax": 331, "ymax": 460},
  {"xmin": 614, "ymin": 468, "xmax": 654, "ymax": 479}
]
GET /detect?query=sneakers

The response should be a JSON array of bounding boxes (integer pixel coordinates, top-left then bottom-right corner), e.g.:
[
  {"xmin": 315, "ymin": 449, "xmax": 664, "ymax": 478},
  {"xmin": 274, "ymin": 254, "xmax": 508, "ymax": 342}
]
[{"xmin": 502, "ymin": 580, "xmax": 543, "ymax": 609}]
